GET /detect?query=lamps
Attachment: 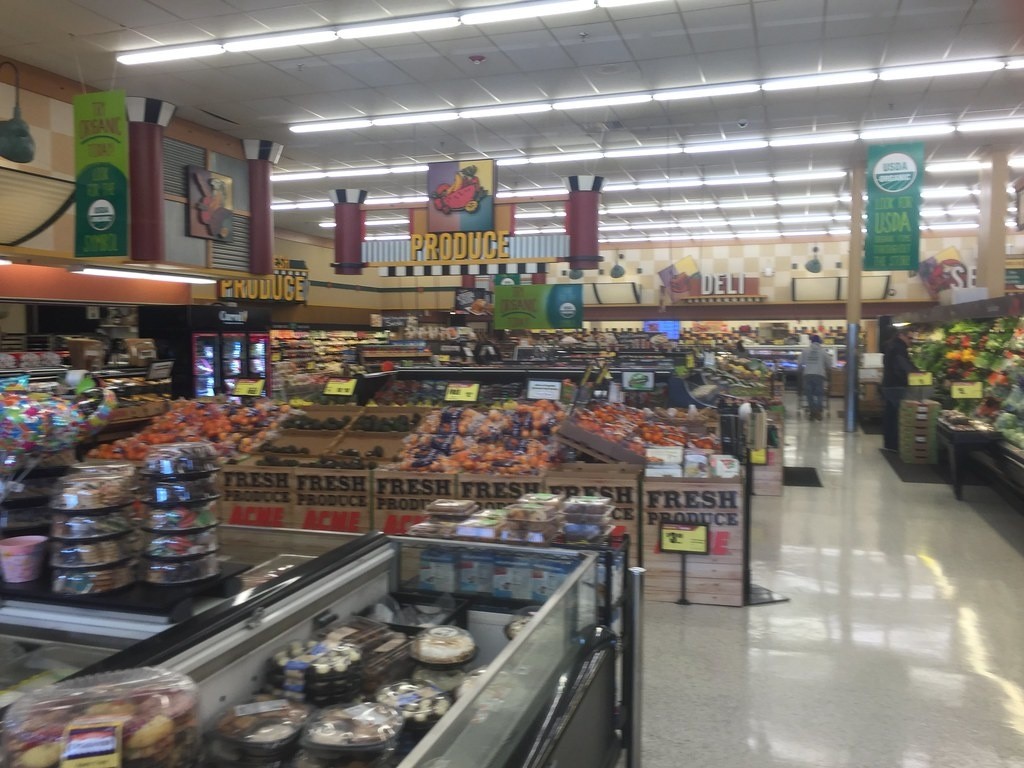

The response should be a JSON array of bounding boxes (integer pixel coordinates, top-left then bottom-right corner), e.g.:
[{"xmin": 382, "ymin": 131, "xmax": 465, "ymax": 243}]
[
  {"xmin": 115, "ymin": 0, "xmax": 662, "ymax": 65},
  {"xmin": 568, "ymin": 267, "xmax": 584, "ymax": 279},
  {"xmin": 268, "ymin": 54, "xmax": 1024, "ymax": 241},
  {"xmin": 0, "ymin": 62, "xmax": 34, "ymax": 164},
  {"xmin": 804, "ymin": 245, "xmax": 822, "ymax": 273},
  {"xmin": 610, "ymin": 251, "xmax": 625, "ymax": 278}
]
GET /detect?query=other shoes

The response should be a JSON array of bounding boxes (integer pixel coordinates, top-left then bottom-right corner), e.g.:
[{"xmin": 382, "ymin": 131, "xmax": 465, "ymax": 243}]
[{"xmin": 883, "ymin": 443, "xmax": 898, "ymax": 452}]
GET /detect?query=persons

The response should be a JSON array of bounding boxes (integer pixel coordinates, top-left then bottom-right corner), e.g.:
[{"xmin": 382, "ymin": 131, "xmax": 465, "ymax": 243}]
[
  {"xmin": 882, "ymin": 328, "xmax": 920, "ymax": 454},
  {"xmin": 796, "ymin": 334, "xmax": 833, "ymax": 420}
]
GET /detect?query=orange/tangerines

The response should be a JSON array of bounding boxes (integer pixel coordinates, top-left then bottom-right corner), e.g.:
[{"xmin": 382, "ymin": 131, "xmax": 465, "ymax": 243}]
[
  {"xmin": 88, "ymin": 401, "xmax": 291, "ymax": 460},
  {"xmin": 575, "ymin": 402, "xmax": 718, "ymax": 451},
  {"xmin": 395, "ymin": 398, "xmax": 566, "ymax": 474}
]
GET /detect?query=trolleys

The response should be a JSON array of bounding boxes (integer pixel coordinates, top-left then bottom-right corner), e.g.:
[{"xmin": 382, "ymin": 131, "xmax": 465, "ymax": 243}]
[{"xmin": 798, "ymin": 365, "xmax": 832, "ymax": 419}]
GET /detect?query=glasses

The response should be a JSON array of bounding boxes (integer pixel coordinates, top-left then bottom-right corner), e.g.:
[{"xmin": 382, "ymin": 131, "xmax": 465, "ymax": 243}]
[{"xmin": 905, "ymin": 335, "xmax": 914, "ymax": 340}]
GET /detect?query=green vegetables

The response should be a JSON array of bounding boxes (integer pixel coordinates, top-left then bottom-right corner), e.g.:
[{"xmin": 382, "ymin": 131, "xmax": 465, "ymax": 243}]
[{"xmin": 909, "ymin": 315, "xmax": 1016, "ymax": 416}]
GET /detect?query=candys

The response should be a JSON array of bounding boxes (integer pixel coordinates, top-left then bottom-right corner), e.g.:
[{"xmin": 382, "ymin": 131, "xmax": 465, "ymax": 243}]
[{"xmin": 0, "ymin": 389, "xmax": 116, "ymax": 504}]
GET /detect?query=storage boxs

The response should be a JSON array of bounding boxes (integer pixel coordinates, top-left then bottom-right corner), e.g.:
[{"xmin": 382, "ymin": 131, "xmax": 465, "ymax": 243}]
[
  {"xmin": 60, "ymin": 335, "xmax": 173, "ymax": 421},
  {"xmin": 898, "ymin": 398, "xmax": 940, "ymax": 464},
  {"xmin": 240, "ymin": 407, "xmax": 742, "ymax": 477},
  {"xmin": 856, "ymin": 349, "xmax": 886, "ymax": 419}
]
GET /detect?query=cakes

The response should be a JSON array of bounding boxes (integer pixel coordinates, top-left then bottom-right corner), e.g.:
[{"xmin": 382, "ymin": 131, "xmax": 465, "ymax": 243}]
[
  {"xmin": 0, "ymin": 607, "xmax": 544, "ymax": 768},
  {"xmin": 48, "ymin": 440, "xmax": 223, "ymax": 597}
]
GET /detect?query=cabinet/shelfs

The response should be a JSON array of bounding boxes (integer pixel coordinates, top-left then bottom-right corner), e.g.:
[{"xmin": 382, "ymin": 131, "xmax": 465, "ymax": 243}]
[
  {"xmin": 1, "ymin": 351, "xmax": 176, "ymax": 477},
  {"xmin": 216, "ymin": 466, "xmax": 749, "ymax": 605}
]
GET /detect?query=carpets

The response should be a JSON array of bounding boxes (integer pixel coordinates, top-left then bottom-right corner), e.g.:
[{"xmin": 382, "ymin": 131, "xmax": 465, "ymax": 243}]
[
  {"xmin": 784, "ymin": 467, "xmax": 823, "ymax": 487},
  {"xmin": 881, "ymin": 448, "xmax": 954, "ymax": 483}
]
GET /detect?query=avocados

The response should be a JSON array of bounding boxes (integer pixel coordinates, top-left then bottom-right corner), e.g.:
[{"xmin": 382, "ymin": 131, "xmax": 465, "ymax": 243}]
[{"xmin": 257, "ymin": 413, "xmax": 422, "ymax": 469}]
